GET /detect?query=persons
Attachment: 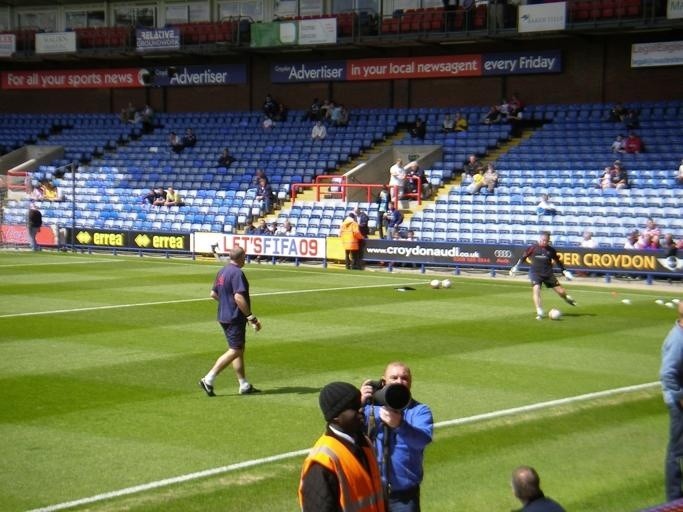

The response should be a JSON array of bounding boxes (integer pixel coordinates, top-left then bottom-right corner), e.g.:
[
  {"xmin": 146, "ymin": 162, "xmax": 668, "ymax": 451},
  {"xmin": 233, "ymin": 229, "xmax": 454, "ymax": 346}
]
[
  {"xmin": 297, "ymin": 382, "xmax": 385, "ymax": 512},
  {"xmin": 463, "ymin": 154, "xmax": 498, "ymax": 194},
  {"xmin": 121, "ymin": 98, "xmax": 154, "ymax": 124},
  {"xmin": 360, "ymin": 361, "xmax": 433, "ymax": 512},
  {"xmin": 440, "ymin": 0, "xmax": 458, "ymax": 31},
  {"xmin": 538, "ymin": 194, "xmax": 554, "ymax": 211},
  {"xmin": 144, "ymin": 187, "xmax": 182, "ymax": 206},
  {"xmin": 506, "ymin": 230, "xmax": 578, "ymax": 321},
  {"xmin": 579, "ymin": 231, "xmax": 599, "ymax": 248},
  {"xmin": 502, "ymin": 465, "xmax": 570, "ymax": 511},
  {"xmin": 607, "ymin": 103, "xmax": 640, "ymax": 129},
  {"xmin": 262, "ymin": 94, "xmax": 349, "ymax": 140},
  {"xmin": 408, "ymin": 112, "xmax": 468, "ymax": 138},
  {"xmin": 338, "ymin": 158, "xmax": 430, "ymax": 270},
  {"xmin": 612, "ymin": 132, "xmax": 642, "ymax": 154},
  {"xmin": 482, "ymin": 93, "xmax": 523, "ymax": 138},
  {"xmin": 217, "ymin": 148, "xmax": 235, "ymax": 168},
  {"xmin": 243, "ymin": 172, "xmax": 296, "ymax": 262},
  {"xmin": 599, "ymin": 160, "xmax": 627, "ymax": 189},
  {"xmin": 169, "ymin": 128, "xmax": 197, "ymax": 154},
  {"xmin": 460, "ymin": 0, "xmax": 475, "ymax": 30},
  {"xmin": 198, "ymin": 247, "xmax": 262, "ymax": 395},
  {"xmin": 26, "ymin": 176, "xmax": 64, "ymax": 250},
  {"xmin": 623, "ymin": 216, "xmax": 682, "ymax": 249},
  {"xmin": 656, "ymin": 298, "xmax": 683, "ymax": 503},
  {"xmin": 488, "ymin": 0, "xmax": 506, "ymax": 28}
]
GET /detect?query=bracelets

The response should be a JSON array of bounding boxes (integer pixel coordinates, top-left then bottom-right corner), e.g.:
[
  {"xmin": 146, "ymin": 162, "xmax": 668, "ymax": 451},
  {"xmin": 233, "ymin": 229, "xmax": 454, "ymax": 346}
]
[{"xmin": 245, "ymin": 314, "xmax": 258, "ymax": 324}]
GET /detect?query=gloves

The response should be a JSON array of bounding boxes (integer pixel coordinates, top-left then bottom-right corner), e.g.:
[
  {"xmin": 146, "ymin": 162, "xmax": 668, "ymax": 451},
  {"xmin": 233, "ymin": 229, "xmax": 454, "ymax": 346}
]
[
  {"xmin": 246, "ymin": 313, "xmax": 262, "ymax": 332},
  {"xmin": 509, "ymin": 265, "xmax": 518, "ymax": 277},
  {"xmin": 563, "ymin": 270, "xmax": 575, "ymax": 281}
]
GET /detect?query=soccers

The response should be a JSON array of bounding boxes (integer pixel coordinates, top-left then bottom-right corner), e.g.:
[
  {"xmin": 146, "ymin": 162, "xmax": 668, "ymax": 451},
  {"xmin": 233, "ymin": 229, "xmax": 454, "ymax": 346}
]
[
  {"xmin": 443, "ymin": 279, "xmax": 451, "ymax": 288},
  {"xmin": 430, "ymin": 280, "xmax": 440, "ymax": 288},
  {"xmin": 549, "ymin": 309, "xmax": 560, "ymax": 320}
]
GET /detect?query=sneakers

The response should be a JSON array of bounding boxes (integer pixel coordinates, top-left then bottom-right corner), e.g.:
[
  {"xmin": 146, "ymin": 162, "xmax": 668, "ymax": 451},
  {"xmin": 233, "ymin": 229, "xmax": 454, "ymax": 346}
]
[
  {"xmin": 535, "ymin": 313, "xmax": 546, "ymax": 321},
  {"xmin": 567, "ymin": 296, "xmax": 576, "ymax": 306},
  {"xmin": 198, "ymin": 377, "xmax": 217, "ymax": 397},
  {"xmin": 239, "ymin": 381, "xmax": 262, "ymax": 394}
]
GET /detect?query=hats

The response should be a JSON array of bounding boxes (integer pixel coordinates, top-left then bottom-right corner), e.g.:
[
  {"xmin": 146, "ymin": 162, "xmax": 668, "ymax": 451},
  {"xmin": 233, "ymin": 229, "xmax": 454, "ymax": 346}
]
[
  {"xmin": 319, "ymin": 382, "xmax": 361, "ymax": 421},
  {"xmin": 613, "ymin": 159, "xmax": 622, "ymax": 166}
]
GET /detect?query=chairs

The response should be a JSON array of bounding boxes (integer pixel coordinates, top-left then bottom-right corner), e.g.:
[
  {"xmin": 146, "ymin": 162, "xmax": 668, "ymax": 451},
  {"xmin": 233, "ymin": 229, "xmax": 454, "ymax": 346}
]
[
  {"xmin": 0, "ymin": 99, "xmax": 682, "ymax": 256},
  {"xmin": 1, "ymin": 0, "xmax": 663, "ymax": 56}
]
